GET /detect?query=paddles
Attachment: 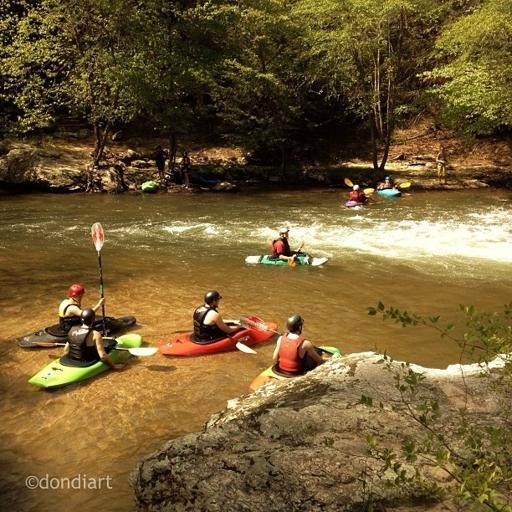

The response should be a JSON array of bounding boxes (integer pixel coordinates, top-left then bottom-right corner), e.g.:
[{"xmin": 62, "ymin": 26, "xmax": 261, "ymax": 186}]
[
  {"xmin": 288, "ymin": 240, "xmax": 305, "ymax": 268},
  {"xmin": 344, "ymin": 177, "xmax": 376, "ymax": 201},
  {"xmin": 91, "ymin": 222, "xmax": 107, "ymax": 337},
  {"xmin": 240, "ymin": 314, "xmax": 341, "ymax": 358},
  {"xmin": 363, "ymin": 182, "xmax": 412, "ymax": 195},
  {"xmin": 223, "ymin": 333, "xmax": 258, "ymax": 355},
  {"xmin": 32, "ymin": 342, "xmax": 159, "ymax": 357}
]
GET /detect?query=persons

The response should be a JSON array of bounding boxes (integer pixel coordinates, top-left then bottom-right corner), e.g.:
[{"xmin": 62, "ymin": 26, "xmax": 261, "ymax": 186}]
[
  {"xmin": 62, "ymin": 306, "xmax": 126, "ymax": 371},
  {"xmin": 57, "ymin": 284, "xmax": 105, "ymax": 330},
  {"xmin": 178, "ymin": 151, "xmax": 192, "ymax": 189},
  {"xmin": 151, "ymin": 144, "xmax": 168, "ymax": 180},
  {"xmin": 375, "ymin": 176, "xmax": 393, "ymax": 190},
  {"xmin": 435, "ymin": 146, "xmax": 451, "ymax": 187},
  {"xmin": 271, "ymin": 313, "xmax": 328, "ymax": 377},
  {"xmin": 269, "ymin": 228, "xmax": 303, "ymax": 263},
  {"xmin": 191, "ymin": 288, "xmax": 245, "ymax": 342},
  {"xmin": 348, "ymin": 183, "xmax": 367, "ymax": 203}
]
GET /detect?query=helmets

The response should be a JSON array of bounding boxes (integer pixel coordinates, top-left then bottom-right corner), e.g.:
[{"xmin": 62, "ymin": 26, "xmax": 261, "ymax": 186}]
[
  {"xmin": 81, "ymin": 308, "xmax": 95, "ymax": 325},
  {"xmin": 385, "ymin": 177, "xmax": 390, "ymax": 180},
  {"xmin": 204, "ymin": 290, "xmax": 222, "ymax": 305},
  {"xmin": 279, "ymin": 228, "xmax": 290, "ymax": 236},
  {"xmin": 287, "ymin": 313, "xmax": 304, "ymax": 332},
  {"xmin": 353, "ymin": 185, "xmax": 359, "ymax": 191},
  {"xmin": 67, "ymin": 284, "xmax": 85, "ymax": 297}
]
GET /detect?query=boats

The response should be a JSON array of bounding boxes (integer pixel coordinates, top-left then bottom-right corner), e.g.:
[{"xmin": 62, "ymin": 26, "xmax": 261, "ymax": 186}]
[
  {"xmin": 345, "ymin": 199, "xmax": 367, "ymax": 208},
  {"xmin": 248, "ymin": 346, "xmax": 340, "ymax": 393},
  {"xmin": 142, "ymin": 181, "xmax": 158, "ymax": 192},
  {"xmin": 245, "ymin": 256, "xmax": 328, "ymax": 268},
  {"xmin": 376, "ymin": 186, "xmax": 402, "ymax": 198},
  {"xmin": 16, "ymin": 317, "xmax": 136, "ymax": 348},
  {"xmin": 158, "ymin": 323, "xmax": 277, "ymax": 357},
  {"xmin": 28, "ymin": 335, "xmax": 142, "ymax": 389}
]
